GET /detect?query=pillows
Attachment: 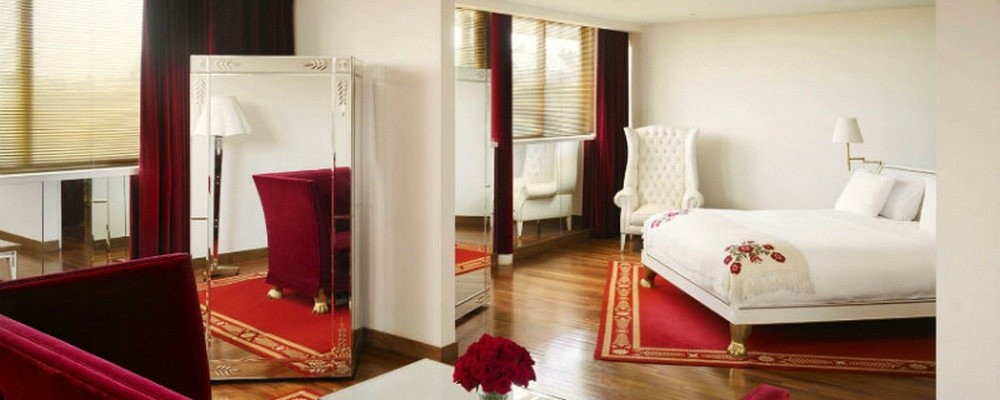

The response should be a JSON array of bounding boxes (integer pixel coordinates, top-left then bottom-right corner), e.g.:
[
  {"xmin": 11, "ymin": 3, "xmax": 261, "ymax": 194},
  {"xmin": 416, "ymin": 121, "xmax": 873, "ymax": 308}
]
[
  {"xmin": 881, "ymin": 180, "xmax": 926, "ymax": 221},
  {"xmin": 835, "ymin": 170, "xmax": 893, "ymax": 218}
]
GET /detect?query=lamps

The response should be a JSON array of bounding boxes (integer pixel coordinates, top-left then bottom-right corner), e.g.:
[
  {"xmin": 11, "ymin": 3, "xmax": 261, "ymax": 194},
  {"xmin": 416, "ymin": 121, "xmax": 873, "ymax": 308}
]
[
  {"xmin": 832, "ymin": 117, "xmax": 882, "ymax": 171},
  {"xmin": 195, "ymin": 94, "xmax": 254, "ymax": 277}
]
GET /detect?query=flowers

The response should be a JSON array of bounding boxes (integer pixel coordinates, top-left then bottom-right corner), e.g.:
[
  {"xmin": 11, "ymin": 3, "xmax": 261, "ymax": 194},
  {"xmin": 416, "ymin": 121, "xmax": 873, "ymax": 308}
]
[{"xmin": 454, "ymin": 332, "xmax": 537, "ymax": 394}]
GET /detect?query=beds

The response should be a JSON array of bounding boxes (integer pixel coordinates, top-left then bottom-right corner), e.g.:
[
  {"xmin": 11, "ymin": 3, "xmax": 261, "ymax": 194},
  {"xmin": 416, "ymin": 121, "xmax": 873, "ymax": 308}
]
[{"xmin": 639, "ymin": 165, "xmax": 937, "ymax": 360}]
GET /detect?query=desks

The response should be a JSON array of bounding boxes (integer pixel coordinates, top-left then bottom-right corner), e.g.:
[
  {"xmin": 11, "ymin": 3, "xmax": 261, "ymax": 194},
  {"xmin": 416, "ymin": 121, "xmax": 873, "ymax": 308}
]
[{"xmin": 318, "ymin": 358, "xmax": 565, "ymax": 400}]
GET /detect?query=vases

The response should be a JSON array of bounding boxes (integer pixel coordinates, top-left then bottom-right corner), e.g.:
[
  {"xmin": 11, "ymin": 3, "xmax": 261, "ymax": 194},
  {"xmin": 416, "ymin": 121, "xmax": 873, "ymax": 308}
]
[{"xmin": 480, "ymin": 388, "xmax": 510, "ymax": 400}]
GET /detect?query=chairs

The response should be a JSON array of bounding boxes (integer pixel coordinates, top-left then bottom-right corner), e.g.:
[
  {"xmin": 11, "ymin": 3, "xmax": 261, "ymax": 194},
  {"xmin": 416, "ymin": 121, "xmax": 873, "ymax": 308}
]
[
  {"xmin": 613, "ymin": 125, "xmax": 704, "ymax": 253},
  {"xmin": 253, "ymin": 166, "xmax": 352, "ymax": 312},
  {"xmin": 0, "ymin": 238, "xmax": 212, "ymax": 400},
  {"xmin": 489, "ymin": 138, "xmax": 583, "ymax": 238}
]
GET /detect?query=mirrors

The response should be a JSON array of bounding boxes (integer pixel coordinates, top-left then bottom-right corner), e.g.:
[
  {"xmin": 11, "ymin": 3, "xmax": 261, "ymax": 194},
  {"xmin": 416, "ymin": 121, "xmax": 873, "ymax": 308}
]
[
  {"xmin": 453, "ymin": 67, "xmax": 494, "ymax": 321},
  {"xmin": 188, "ymin": 53, "xmax": 354, "ymax": 376}
]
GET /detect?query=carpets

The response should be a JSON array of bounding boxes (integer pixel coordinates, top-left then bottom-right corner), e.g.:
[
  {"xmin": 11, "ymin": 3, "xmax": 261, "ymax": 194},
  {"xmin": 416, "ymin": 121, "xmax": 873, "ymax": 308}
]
[
  {"xmin": 269, "ymin": 388, "xmax": 325, "ymax": 400},
  {"xmin": 195, "ymin": 270, "xmax": 350, "ymax": 374},
  {"xmin": 454, "ymin": 241, "xmax": 492, "ymax": 274},
  {"xmin": 594, "ymin": 261, "xmax": 936, "ymax": 375}
]
